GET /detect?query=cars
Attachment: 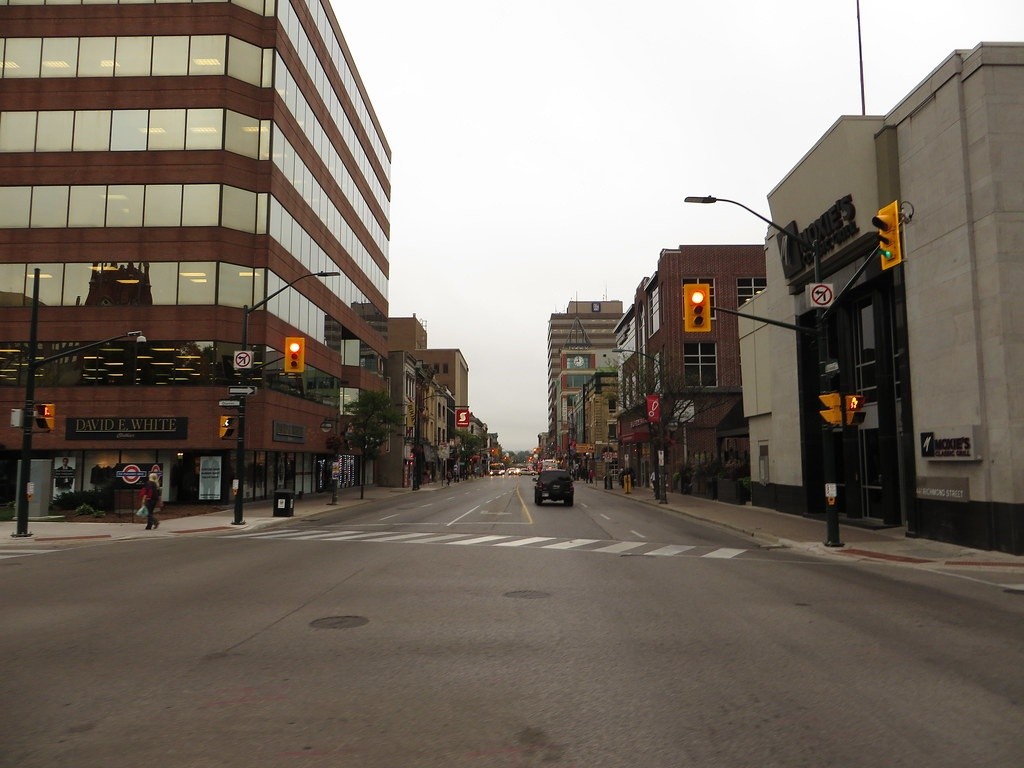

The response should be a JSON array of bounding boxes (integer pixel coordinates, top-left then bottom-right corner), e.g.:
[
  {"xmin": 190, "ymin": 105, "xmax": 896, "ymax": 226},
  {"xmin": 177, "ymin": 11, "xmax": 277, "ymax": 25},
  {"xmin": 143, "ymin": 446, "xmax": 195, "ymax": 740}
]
[{"xmin": 490, "ymin": 458, "xmax": 557, "ymax": 476}]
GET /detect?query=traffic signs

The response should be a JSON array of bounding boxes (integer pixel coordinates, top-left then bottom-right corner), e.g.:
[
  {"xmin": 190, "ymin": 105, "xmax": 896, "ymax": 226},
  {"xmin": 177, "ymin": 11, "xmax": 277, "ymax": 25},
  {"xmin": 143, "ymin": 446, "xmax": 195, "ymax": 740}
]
[
  {"xmin": 219, "ymin": 398, "xmax": 247, "ymax": 411},
  {"xmin": 227, "ymin": 385, "xmax": 258, "ymax": 396}
]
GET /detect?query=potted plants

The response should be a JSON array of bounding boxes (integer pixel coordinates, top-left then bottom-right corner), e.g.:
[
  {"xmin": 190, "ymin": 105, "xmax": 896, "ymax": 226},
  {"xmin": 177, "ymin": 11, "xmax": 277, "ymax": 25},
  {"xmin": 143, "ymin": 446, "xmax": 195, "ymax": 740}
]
[{"xmin": 672, "ymin": 461, "xmax": 751, "ymax": 505}]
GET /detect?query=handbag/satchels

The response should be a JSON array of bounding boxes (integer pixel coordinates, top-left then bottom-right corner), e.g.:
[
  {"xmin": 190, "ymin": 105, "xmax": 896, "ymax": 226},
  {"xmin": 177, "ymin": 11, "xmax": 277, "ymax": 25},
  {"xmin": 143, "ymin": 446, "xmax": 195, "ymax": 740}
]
[{"xmin": 136, "ymin": 506, "xmax": 149, "ymax": 517}]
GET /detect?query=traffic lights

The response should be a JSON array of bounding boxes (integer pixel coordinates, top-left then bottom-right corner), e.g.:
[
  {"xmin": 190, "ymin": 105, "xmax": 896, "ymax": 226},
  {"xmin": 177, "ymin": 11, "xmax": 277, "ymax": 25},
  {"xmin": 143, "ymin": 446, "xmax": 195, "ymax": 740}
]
[
  {"xmin": 283, "ymin": 335, "xmax": 304, "ymax": 376},
  {"xmin": 34, "ymin": 401, "xmax": 56, "ymax": 431},
  {"xmin": 871, "ymin": 199, "xmax": 904, "ymax": 272},
  {"xmin": 845, "ymin": 393, "xmax": 868, "ymax": 425},
  {"xmin": 684, "ymin": 282, "xmax": 714, "ymax": 333},
  {"xmin": 818, "ymin": 390, "xmax": 842, "ymax": 425},
  {"xmin": 220, "ymin": 412, "xmax": 236, "ymax": 441}
]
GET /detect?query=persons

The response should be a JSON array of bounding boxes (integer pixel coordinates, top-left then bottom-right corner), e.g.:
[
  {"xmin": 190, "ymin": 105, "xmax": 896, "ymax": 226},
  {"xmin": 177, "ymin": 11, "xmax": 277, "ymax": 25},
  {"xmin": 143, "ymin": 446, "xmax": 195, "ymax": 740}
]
[
  {"xmin": 138, "ymin": 473, "xmax": 161, "ymax": 530},
  {"xmin": 619, "ymin": 468, "xmax": 635, "ymax": 488},
  {"xmin": 201, "ymin": 457, "xmax": 219, "ymax": 499},
  {"xmin": 446, "ymin": 472, "xmax": 451, "ymax": 486},
  {"xmin": 55, "ymin": 457, "xmax": 73, "ymax": 493},
  {"xmin": 651, "ymin": 472, "xmax": 661, "ymax": 493},
  {"xmin": 572, "ymin": 469, "xmax": 593, "ymax": 484}
]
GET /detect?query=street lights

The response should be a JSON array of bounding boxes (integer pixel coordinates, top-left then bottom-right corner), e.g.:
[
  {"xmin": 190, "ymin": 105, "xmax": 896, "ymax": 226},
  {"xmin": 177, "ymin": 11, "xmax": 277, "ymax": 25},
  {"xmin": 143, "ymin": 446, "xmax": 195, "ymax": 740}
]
[
  {"xmin": 12, "ymin": 325, "xmax": 146, "ymax": 538},
  {"xmin": 684, "ymin": 194, "xmax": 843, "ymax": 551},
  {"xmin": 227, "ymin": 271, "xmax": 343, "ymax": 525},
  {"xmin": 610, "ymin": 346, "xmax": 669, "ymax": 504},
  {"xmin": 414, "ymin": 393, "xmax": 449, "ymax": 488}
]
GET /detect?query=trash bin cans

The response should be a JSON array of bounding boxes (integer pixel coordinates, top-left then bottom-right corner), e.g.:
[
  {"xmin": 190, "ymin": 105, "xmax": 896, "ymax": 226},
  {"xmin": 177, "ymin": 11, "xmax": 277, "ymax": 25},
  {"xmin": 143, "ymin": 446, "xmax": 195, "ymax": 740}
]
[
  {"xmin": 603, "ymin": 475, "xmax": 613, "ymax": 490},
  {"xmin": 454, "ymin": 474, "xmax": 459, "ymax": 482},
  {"xmin": 273, "ymin": 488, "xmax": 295, "ymax": 517}
]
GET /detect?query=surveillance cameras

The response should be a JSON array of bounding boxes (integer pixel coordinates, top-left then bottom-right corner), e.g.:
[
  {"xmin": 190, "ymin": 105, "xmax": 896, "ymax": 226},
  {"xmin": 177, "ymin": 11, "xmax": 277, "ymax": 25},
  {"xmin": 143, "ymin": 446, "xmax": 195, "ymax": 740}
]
[{"xmin": 137, "ymin": 336, "xmax": 147, "ymax": 345}]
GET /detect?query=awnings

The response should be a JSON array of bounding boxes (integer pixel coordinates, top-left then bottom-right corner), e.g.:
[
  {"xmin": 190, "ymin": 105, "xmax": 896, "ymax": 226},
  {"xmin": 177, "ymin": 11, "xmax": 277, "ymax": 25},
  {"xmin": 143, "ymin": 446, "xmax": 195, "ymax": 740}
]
[{"xmin": 405, "ymin": 444, "xmax": 438, "ymax": 462}]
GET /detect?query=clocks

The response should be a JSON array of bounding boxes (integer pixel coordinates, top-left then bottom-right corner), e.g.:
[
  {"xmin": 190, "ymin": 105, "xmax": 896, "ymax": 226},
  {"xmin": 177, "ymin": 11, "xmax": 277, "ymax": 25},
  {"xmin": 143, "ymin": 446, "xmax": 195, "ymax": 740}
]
[{"xmin": 573, "ymin": 356, "xmax": 584, "ymax": 367}]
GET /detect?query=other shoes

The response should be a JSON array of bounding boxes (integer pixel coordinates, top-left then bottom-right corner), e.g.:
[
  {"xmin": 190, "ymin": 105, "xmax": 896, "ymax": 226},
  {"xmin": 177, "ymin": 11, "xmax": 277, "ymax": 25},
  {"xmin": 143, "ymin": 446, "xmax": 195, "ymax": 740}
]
[
  {"xmin": 145, "ymin": 526, "xmax": 151, "ymax": 530},
  {"xmin": 154, "ymin": 521, "xmax": 160, "ymax": 529}
]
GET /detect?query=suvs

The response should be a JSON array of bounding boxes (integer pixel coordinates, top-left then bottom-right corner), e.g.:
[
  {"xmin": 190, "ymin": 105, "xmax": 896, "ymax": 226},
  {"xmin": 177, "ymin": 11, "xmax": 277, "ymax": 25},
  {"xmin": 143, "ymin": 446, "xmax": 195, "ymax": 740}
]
[{"xmin": 530, "ymin": 469, "xmax": 574, "ymax": 507}]
